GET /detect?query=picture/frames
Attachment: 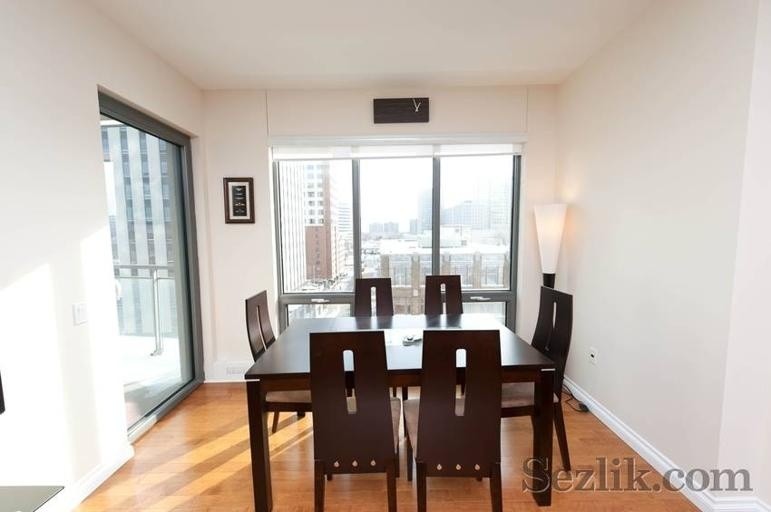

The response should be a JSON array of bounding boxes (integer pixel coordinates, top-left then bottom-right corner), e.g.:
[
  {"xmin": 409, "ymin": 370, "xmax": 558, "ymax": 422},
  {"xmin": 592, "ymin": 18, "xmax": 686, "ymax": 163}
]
[{"xmin": 223, "ymin": 177, "xmax": 255, "ymax": 224}]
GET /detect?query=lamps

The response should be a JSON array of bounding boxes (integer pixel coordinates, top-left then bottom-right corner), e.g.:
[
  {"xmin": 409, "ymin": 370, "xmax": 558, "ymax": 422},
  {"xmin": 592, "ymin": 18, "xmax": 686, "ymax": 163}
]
[{"xmin": 534, "ymin": 203, "xmax": 566, "ymax": 289}]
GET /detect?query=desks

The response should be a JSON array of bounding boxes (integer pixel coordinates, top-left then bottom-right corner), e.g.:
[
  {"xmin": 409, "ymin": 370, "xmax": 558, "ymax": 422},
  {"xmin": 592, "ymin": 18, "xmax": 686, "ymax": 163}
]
[{"xmin": 244, "ymin": 315, "xmax": 557, "ymax": 512}]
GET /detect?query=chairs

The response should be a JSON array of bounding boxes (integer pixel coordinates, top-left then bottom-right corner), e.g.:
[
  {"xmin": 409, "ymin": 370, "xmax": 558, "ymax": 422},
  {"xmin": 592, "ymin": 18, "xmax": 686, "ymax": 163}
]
[
  {"xmin": 402, "ymin": 331, "xmax": 502, "ymax": 511},
  {"xmin": 424, "ymin": 274, "xmax": 463, "ymax": 314},
  {"xmin": 309, "ymin": 330, "xmax": 413, "ymax": 512},
  {"xmin": 346, "ymin": 278, "xmax": 408, "ymax": 402},
  {"xmin": 461, "ymin": 286, "xmax": 573, "ymax": 482},
  {"xmin": 245, "ymin": 290, "xmax": 304, "ymax": 431},
  {"xmin": 374, "ymin": 98, "xmax": 429, "ymax": 124}
]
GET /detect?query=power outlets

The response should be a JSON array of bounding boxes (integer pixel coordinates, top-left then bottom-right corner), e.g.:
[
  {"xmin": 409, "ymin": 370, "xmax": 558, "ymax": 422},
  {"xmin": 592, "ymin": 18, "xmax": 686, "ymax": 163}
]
[{"xmin": 587, "ymin": 347, "xmax": 597, "ymax": 363}]
